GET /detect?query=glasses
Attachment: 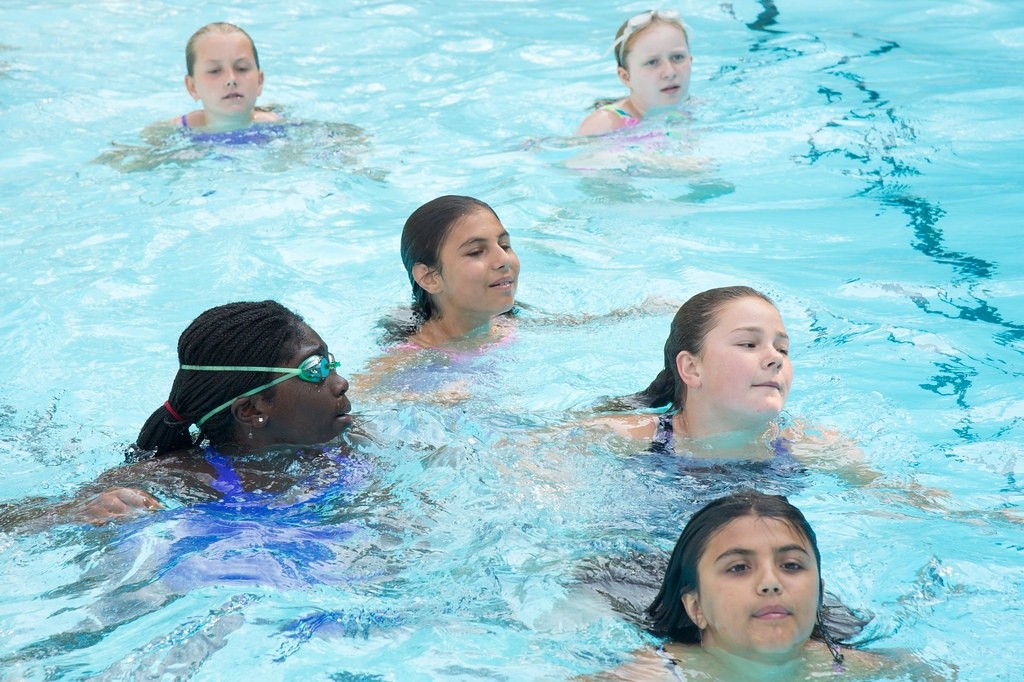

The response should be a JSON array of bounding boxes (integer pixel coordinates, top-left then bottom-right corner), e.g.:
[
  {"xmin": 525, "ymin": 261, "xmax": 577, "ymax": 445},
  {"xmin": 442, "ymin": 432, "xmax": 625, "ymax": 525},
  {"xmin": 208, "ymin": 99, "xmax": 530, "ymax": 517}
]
[
  {"xmin": 297, "ymin": 353, "xmax": 340, "ymax": 384},
  {"xmin": 619, "ymin": 11, "xmax": 681, "ymax": 66}
]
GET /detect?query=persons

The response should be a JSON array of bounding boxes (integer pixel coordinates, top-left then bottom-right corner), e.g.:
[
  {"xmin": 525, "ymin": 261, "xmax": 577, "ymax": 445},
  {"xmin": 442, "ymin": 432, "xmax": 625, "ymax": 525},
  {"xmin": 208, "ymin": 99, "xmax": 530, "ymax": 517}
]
[
  {"xmin": 650, "ymin": 491, "xmax": 824, "ymax": 665},
  {"xmin": 649, "ymin": 286, "xmax": 793, "ymax": 436},
  {"xmin": 400, "ymin": 195, "xmax": 520, "ymax": 342},
  {"xmin": 175, "ymin": 23, "xmax": 273, "ymax": 125},
  {"xmin": 138, "ymin": 300, "xmax": 352, "ymax": 455},
  {"xmin": 578, "ymin": 10, "xmax": 693, "ymax": 133}
]
